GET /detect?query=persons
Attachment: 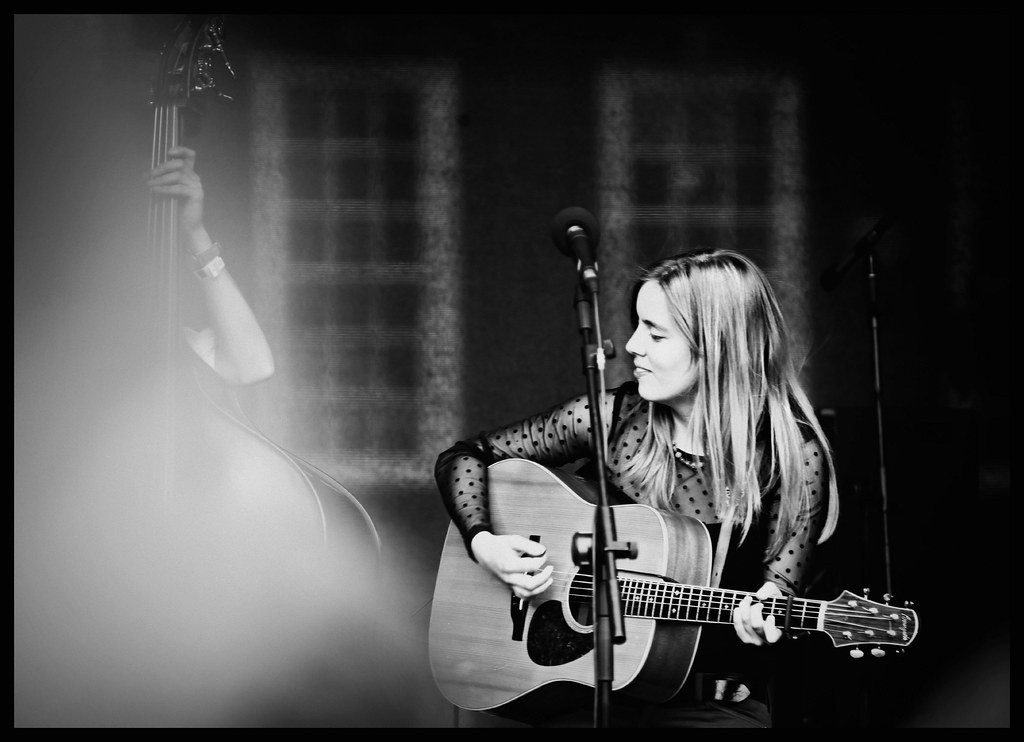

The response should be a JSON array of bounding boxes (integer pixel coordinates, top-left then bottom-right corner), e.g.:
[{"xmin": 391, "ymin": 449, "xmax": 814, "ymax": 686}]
[
  {"xmin": 433, "ymin": 247, "xmax": 840, "ymax": 729},
  {"xmin": 148, "ymin": 144, "xmax": 278, "ymax": 389}
]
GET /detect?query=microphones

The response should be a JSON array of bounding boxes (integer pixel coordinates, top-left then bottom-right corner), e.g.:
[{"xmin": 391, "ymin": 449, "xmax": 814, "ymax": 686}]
[{"xmin": 554, "ymin": 208, "xmax": 600, "ymax": 293}]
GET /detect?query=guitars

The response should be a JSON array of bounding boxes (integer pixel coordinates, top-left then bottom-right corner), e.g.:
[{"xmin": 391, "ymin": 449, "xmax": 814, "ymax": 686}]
[{"xmin": 425, "ymin": 457, "xmax": 921, "ymax": 714}]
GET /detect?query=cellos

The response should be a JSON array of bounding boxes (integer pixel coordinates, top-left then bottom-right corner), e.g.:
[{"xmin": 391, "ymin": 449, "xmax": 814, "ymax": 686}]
[{"xmin": 139, "ymin": 20, "xmax": 384, "ymax": 566}]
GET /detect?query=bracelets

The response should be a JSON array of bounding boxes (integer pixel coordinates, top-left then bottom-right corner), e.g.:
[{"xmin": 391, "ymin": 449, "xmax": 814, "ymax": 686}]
[{"xmin": 186, "ymin": 243, "xmax": 225, "ymax": 282}]
